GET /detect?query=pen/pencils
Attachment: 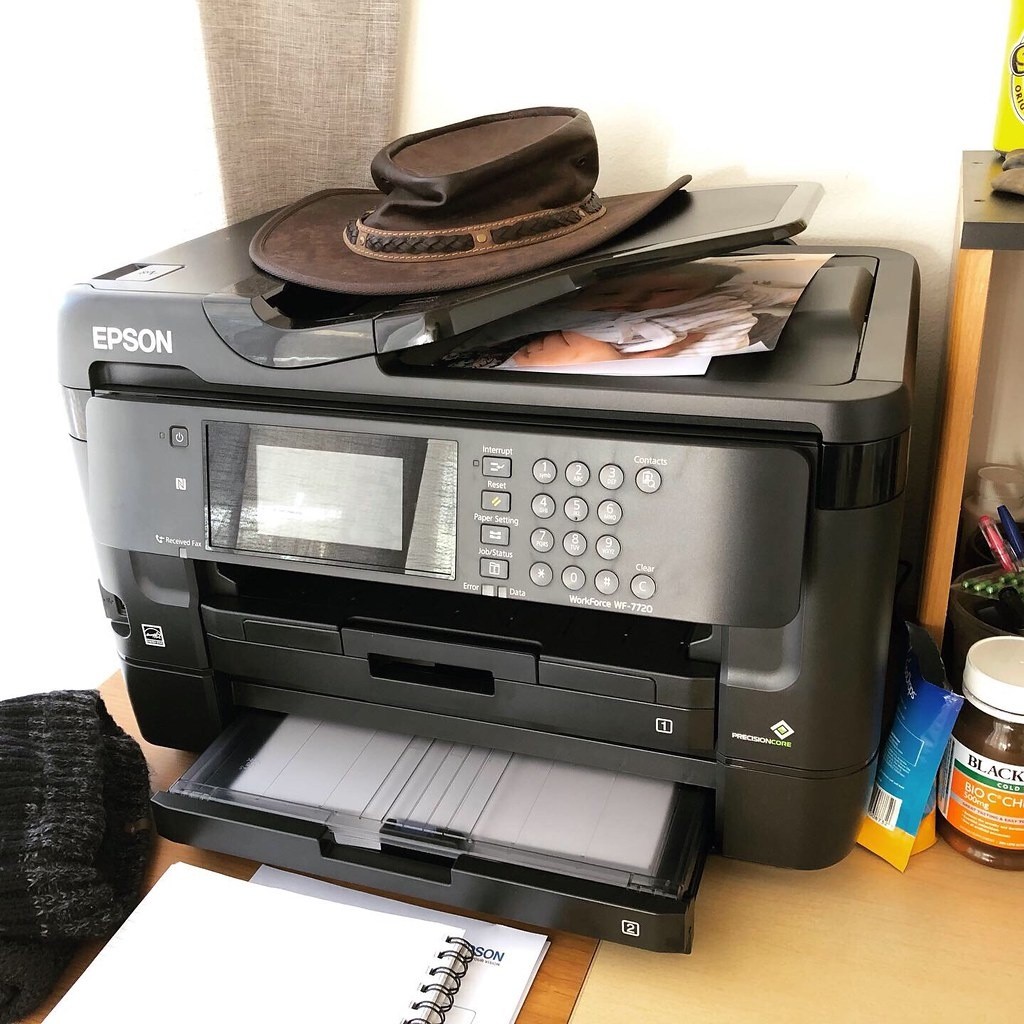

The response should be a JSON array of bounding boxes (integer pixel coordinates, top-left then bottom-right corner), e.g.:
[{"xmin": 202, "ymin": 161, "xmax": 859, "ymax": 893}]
[{"xmin": 961, "ymin": 502, "xmax": 1024, "ymax": 602}]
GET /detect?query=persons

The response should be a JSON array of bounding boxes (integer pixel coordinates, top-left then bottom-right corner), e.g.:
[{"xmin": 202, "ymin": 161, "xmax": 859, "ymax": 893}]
[{"xmin": 512, "ymin": 263, "xmax": 756, "ymax": 365}]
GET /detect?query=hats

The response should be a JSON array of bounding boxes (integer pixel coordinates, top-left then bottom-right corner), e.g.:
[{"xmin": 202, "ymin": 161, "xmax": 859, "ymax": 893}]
[
  {"xmin": 0, "ymin": 689, "xmax": 153, "ymax": 1023},
  {"xmin": 248, "ymin": 106, "xmax": 692, "ymax": 295}
]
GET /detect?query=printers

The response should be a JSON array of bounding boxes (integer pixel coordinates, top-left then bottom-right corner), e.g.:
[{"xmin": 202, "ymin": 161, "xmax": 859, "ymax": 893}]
[{"xmin": 59, "ymin": 182, "xmax": 922, "ymax": 956}]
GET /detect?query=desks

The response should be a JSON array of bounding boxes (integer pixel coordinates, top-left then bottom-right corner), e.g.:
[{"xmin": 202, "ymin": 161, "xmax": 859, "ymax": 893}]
[{"xmin": 20, "ymin": 666, "xmax": 1024, "ymax": 1024}]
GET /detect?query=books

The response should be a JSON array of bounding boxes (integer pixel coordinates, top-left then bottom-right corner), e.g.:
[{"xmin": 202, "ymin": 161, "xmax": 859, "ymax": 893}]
[{"xmin": 41, "ymin": 861, "xmax": 475, "ymax": 1024}]
